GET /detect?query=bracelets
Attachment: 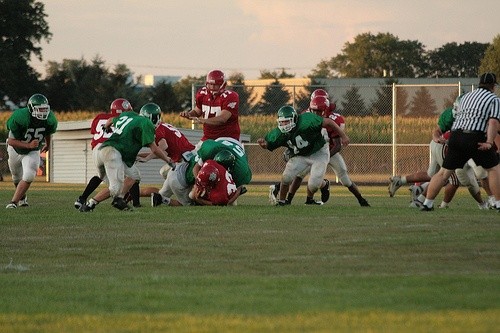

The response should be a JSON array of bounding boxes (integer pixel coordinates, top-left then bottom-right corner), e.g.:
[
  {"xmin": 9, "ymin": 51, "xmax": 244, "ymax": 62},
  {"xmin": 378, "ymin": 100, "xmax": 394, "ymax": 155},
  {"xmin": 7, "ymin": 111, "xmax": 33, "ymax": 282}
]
[
  {"xmin": 486, "ymin": 142, "xmax": 492, "ymax": 146},
  {"xmin": 168, "ymin": 160, "xmax": 176, "ymax": 168}
]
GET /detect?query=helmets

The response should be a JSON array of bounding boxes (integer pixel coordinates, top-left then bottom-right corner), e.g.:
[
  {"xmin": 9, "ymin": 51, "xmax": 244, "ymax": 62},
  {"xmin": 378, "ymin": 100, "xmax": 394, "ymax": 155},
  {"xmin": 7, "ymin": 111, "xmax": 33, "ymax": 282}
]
[
  {"xmin": 205, "ymin": 70, "xmax": 227, "ymax": 95},
  {"xmin": 140, "ymin": 103, "xmax": 161, "ymax": 130},
  {"xmin": 110, "ymin": 98, "xmax": 132, "ymax": 116},
  {"xmin": 310, "ymin": 97, "xmax": 330, "ymax": 117},
  {"xmin": 27, "ymin": 94, "xmax": 50, "ymax": 121},
  {"xmin": 196, "ymin": 165, "xmax": 219, "ymax": 189},
  {"xmin": 311, "ymin": 89, "xmax": 328, "ymax": 99},
  {"xmin": 213, "ymin": 150, "xmax": 235, "ymax": 173},
  {"xmin": 277, "ymin": 106, "xmax": 297, "ymax": 133}
]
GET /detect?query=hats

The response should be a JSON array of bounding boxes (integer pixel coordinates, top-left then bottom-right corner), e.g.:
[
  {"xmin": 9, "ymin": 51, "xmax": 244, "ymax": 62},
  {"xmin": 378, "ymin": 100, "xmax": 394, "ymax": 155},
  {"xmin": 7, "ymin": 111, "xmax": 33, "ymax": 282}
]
[{"xmin": 480, "ymin": 73, "xmax": 498, "ymax": 85}]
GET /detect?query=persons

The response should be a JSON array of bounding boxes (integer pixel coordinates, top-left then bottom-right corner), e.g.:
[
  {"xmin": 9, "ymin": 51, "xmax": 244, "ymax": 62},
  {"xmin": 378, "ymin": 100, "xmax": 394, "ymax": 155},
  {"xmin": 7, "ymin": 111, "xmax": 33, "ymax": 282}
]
[
  {"xmin": 4, "ymin": 94, "xmax": 58, "ymax": 209},
  {"xmin": 74, "ymin": 98, "xmax": 176, "ymax": 211},
  {"xmin": 268, "ymin": 89, "xmax": 372, "ymax": 207},
  {"xmin": 136, "ymin": 122, "xmax": 195, "ymax": 180},
  {"xmin": 420, "ymin": 72, "xmax": 500, "ymax": 211},
  {"xmin": 179, "ymin": 69, "xmax": 241, "ymax": 150},
  {"xmin": 388, "ymin": 93, "xmax": 500, "ymax": 209},
  {"xmin": 257, "ymin": 106, "xmax": 350, "ymax": 208},
  {"xmin": 139, "ymin": 137, "xmax": 252, "ymax": 206}
]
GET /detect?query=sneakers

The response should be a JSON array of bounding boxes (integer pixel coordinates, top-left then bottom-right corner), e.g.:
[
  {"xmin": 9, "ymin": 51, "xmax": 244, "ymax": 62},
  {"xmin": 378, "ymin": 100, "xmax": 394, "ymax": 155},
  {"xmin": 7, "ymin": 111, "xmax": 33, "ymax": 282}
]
[
  {"xmin": 409, "ymin": 184, "xmax": 423, "ymax": 201},
  {"xmin": 439, "ymin": 201, "xmax": 449, "ymax": 209},
  {"xmin": 320, "ymin": 179, "xmax": 330, "ymax": 203},
  {"xmin": 388, "ymin": 176, "xmax": 402, "ymax": 197},
  {"xmin": 74, "ymin": 198, "xmax": 82, "ymax": 209},
  {"xmin": 18, "ymin": 194, "xmax": 29, "ymax": 207},
  {"xmin": 277, "ymin": 200, "xmax": 285, "ymax": 206},
  {"xmin": 133, "ymin": 201, "xmax": 141, "ymax": 207},
  {"xmin": 479, "ymin": 196, "xmax": 500, "ymax": 209},
  {"xmin": 409, "ymin": 199, "xmax": 423, "ymax": 208},
  {"xmin": 285, "ymin": 199, "xmax": 291, "ymax": 205},
  {"xmin": 419, "ymin": 204, "xmax": 434, "ymax": 211},
  {"xmin": 79, "ymin": 201, "xmax": 95, "ymax": 212},
  {"xmin": 361, "ymin": 201, "xmax": 370, "ymax": 206},
  {"xmin": 151, "ymin": 192, "xmax": 162, "ymax": 207},
  {"xmin": 6, "ymin": 203, "xmax": 17, "ymax": 208},
  {"xmin": 305, "ymin": 198, "xmax": 321, "ymax": 205},
  {"xmin": 269, "ymin": 184, "xmax": 278, "ymax": 205},
  {"xmin": 111, "ymin": 197, "xmax": 129, "ymax": 210}
]
